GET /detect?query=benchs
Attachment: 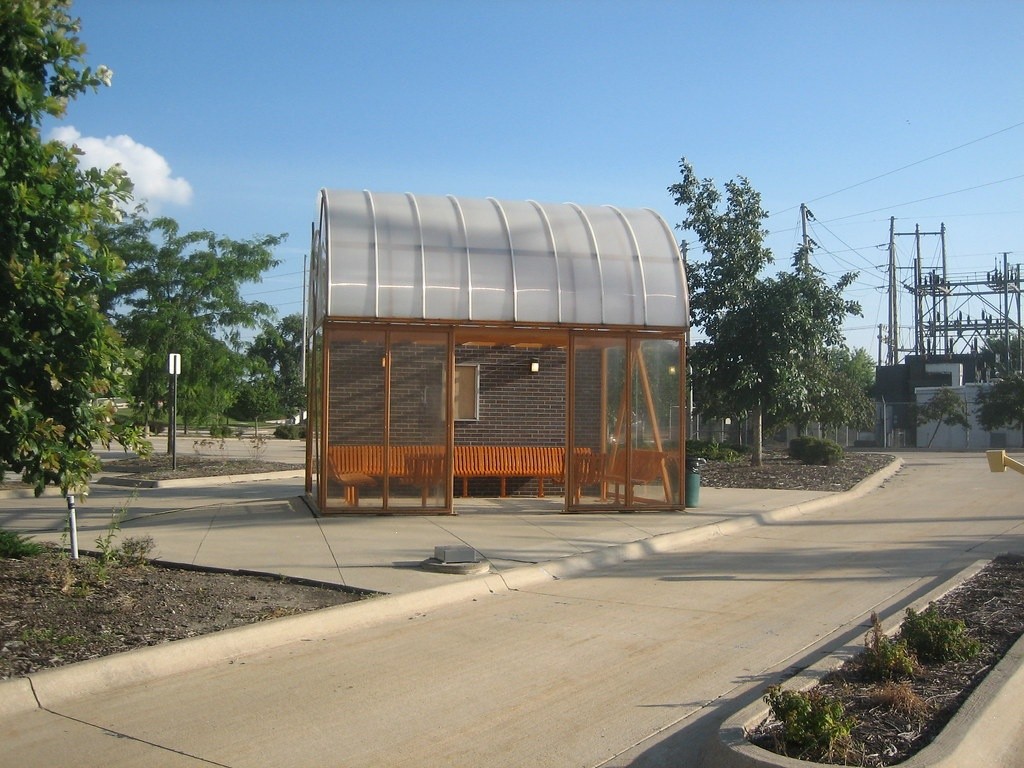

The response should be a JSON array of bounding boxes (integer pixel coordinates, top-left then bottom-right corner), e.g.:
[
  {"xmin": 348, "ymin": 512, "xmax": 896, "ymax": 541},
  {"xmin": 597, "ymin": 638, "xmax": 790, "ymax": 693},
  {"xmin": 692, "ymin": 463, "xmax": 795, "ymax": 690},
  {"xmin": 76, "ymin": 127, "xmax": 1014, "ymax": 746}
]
[
  {"xmin": 328, "ymin": 452, "xmax": 376, "ymax": 508},
  {"xmin": 603, "ymin": 448, "xmax": 668, "ymax": 504},
  {"xmin": 328, "ymin": 444, "xmax": 592, "ymax": 499}
]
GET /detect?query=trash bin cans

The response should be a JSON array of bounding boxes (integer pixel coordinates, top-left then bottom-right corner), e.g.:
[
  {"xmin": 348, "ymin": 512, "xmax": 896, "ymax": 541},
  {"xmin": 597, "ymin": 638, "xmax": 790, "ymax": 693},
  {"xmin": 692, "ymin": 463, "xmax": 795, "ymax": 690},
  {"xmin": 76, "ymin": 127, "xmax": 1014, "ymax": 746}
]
[{"xmin": 665, "ymin": 454, "xmax": 706, "ymax": 509}]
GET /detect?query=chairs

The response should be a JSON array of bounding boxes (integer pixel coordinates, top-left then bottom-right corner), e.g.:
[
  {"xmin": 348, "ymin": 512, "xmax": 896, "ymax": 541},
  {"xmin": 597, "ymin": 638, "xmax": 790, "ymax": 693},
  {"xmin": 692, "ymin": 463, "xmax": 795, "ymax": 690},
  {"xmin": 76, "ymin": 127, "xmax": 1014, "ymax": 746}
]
[
  {"xmin": 398, "ymin": 453, "xmax": 445, "ymax": 507},
  {"xmin": 552, "ymin": 453, "xmax": 607, "ymax": 507}
]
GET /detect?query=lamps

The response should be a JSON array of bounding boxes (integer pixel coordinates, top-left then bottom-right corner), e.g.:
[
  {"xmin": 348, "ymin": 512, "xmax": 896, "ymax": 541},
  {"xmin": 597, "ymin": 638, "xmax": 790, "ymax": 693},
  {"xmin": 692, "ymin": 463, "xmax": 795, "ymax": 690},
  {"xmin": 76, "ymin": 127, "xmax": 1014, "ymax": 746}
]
[{"xmin": 529, "ymin": 357, "xmax": 539, "ymax": 374}]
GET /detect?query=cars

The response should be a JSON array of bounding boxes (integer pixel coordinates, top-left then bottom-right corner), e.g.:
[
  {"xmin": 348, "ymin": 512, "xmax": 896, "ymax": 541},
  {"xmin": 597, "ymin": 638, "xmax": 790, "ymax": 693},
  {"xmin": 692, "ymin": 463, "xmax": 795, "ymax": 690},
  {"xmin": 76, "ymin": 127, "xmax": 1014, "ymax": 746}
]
[{"xmin": 605, "ymin": 410, "xmax": 644, "ymax": 448}]
